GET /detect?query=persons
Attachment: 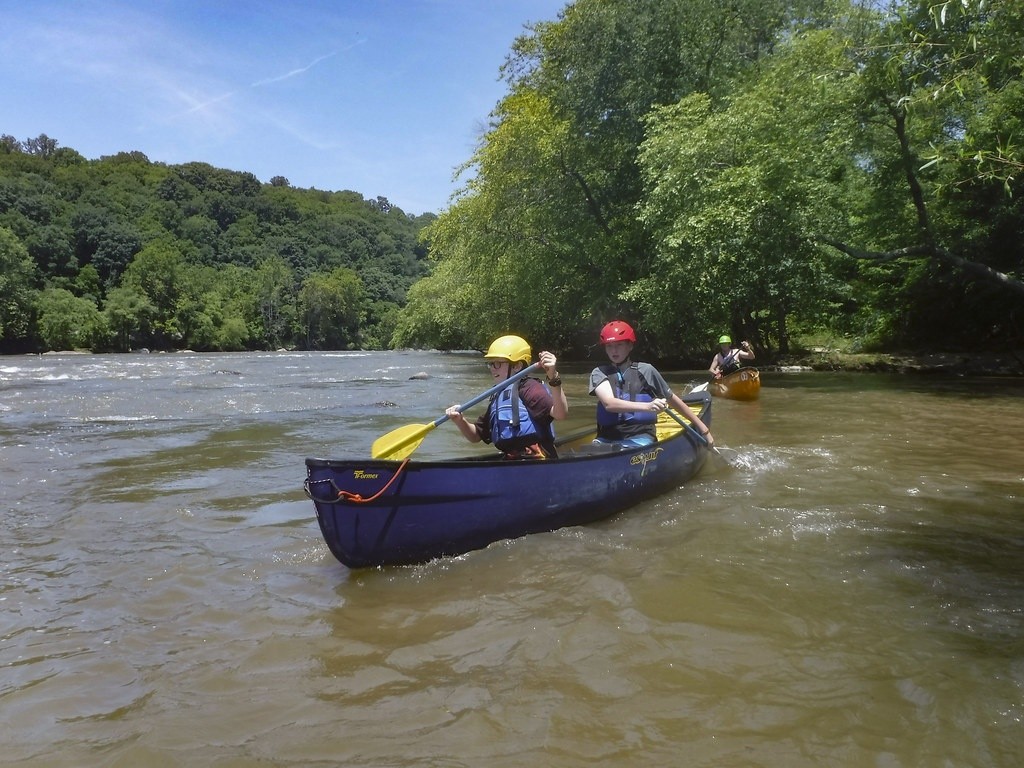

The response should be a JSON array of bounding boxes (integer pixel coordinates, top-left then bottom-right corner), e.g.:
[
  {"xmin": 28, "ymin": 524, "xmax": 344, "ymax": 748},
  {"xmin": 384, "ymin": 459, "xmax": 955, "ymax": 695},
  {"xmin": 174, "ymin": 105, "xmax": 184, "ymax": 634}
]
[
  {"xmin": 709, "ymin": 334, "xmax": 755, "ymax": 380},
  {"xmin": 445, "ymin": 334, "xmax": 568, "ymax": 460},
  {"xmin": 588, "ymin": 320, "xmax": 714, "ymax": 451}
]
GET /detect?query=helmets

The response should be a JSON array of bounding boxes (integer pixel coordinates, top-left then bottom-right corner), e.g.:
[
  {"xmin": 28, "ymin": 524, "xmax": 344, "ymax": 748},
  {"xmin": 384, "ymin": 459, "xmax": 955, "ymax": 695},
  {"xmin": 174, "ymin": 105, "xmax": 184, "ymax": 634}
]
[
  {"xmin": 484, "ymin": 336, "xmax": 532, "ymax": 366},
  {"xmin": 719, "ymin": 335, "xmax": 731, "ymax": 344},
  {"xmin": 600, "ymin": 321, "xmax": 636, "ymax": 346}
]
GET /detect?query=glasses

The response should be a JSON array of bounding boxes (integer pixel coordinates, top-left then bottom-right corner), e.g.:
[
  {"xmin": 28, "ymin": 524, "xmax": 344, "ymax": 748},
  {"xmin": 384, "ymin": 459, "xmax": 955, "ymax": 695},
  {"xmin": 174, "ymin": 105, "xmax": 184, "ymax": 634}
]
[{"xmin": 485, "ymin": 361, "xmax": 509, "ymax": 369}]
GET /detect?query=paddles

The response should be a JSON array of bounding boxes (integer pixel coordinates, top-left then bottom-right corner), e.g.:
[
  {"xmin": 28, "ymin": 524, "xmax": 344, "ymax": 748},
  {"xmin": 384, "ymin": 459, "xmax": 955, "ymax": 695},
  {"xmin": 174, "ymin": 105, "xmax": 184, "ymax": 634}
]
[
  {"xmin": 664, "ymin": 405, "xmax": 739, "ymax": 468},
  {"xmin": 690, "ymin": 346, "xmax": 744, "ymax": 394},
  {"xmin": 370, "ymin": 362, "xmax": 543, "ymax": 460}
]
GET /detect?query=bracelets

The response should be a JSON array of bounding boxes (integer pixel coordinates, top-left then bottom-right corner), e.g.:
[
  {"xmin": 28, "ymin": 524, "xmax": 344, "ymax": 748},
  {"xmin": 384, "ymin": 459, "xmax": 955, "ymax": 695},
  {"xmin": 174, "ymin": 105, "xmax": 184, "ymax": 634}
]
[
  {"xmin": 702, "ymin": 429, "xmax": 710, "ymax": 436},
  {"xmin": 746, "ymin": 346, "xmax": 751, "ymax": 351},
  {"xmin": 545, "ymin": 372, "xmax": 562, "ymax": 387}
]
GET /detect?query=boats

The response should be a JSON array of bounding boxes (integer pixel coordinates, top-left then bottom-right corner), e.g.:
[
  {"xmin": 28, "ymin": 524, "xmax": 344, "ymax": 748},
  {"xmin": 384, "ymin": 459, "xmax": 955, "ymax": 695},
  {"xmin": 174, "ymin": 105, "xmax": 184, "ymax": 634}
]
[
  {"xmin": 707, "ymin": 365, "xmax": 760, "ymax": 401},
  {"xmin": 303, "ymin": 382, "xmax": 711, "ymax": 568}
]
